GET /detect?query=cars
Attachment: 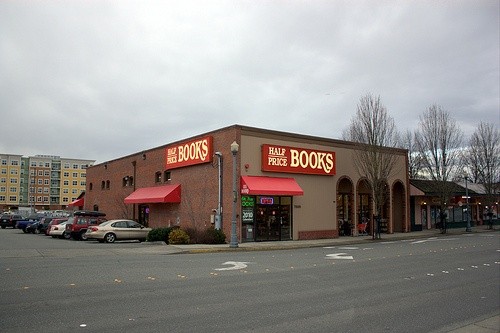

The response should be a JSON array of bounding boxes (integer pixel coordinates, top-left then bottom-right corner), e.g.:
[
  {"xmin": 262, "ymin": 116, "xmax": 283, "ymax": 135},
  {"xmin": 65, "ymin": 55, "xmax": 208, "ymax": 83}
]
[
  {"xmin": 84, "ymin": 219, "xmax": 152, "ymax": 243},
  {"xmin": 15, "ymin": 216, "xmax": 68, "ymax": 234},
  {"xmin": 48, "ymin": 219, "xmax": 87, "ymax": 240}
]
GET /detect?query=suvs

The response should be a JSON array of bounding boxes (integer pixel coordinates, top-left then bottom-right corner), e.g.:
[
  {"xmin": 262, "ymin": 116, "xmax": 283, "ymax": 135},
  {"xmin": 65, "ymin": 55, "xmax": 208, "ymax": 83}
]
[
  {"xmin": 64, "ymin": 211, "xmax": 108, "ymax": 241},
  {"xmin": 0, "ymin": 214, "xmax": 29, "ymax": 229}
]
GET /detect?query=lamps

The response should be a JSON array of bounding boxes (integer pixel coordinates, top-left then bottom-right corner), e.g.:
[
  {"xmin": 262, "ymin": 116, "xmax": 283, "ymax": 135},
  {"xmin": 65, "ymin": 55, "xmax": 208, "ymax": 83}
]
[
  {"xmin": 104, "ymin": 165, "xmax": 107, "ymax": 169},
  {"xmin": 125, "ymin": 176, "xmax": 133, "ymax": 181},
  {"xmin": 142, "ymin": 154, "xmax": 146, "ymax": 160}
]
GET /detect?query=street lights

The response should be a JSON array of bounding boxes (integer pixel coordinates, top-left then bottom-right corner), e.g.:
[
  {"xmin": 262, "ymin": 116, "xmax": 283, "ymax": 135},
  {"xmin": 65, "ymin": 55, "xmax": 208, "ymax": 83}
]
[
  {"xmin": 464, "ymin": 171, "xmax": 472, "ymax": 232},
  {"xmin": 229, "ymin": 140, "xmax": 240, "ymax": 248}
]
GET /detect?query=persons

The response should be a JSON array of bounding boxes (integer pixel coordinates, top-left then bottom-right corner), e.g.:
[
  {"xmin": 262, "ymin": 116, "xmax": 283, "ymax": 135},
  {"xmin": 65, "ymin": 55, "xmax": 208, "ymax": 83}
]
[{"xmin": 373, "ymin": 216, "xmax": 379, "ymax": 240}]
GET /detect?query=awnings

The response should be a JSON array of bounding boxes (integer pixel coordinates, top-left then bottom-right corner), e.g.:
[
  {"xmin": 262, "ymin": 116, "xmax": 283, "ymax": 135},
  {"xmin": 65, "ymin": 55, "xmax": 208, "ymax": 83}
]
[
  {"xmin": 124, "ymin": 184, "xmax": 182, "ymax": 204},
  {"xmin": 69, "ymin": 198, "xmax": 84, "ymax": 207},
  {"xmin": 240, "ymin": 176, "xmax": 304, "ymax": 196}
]
